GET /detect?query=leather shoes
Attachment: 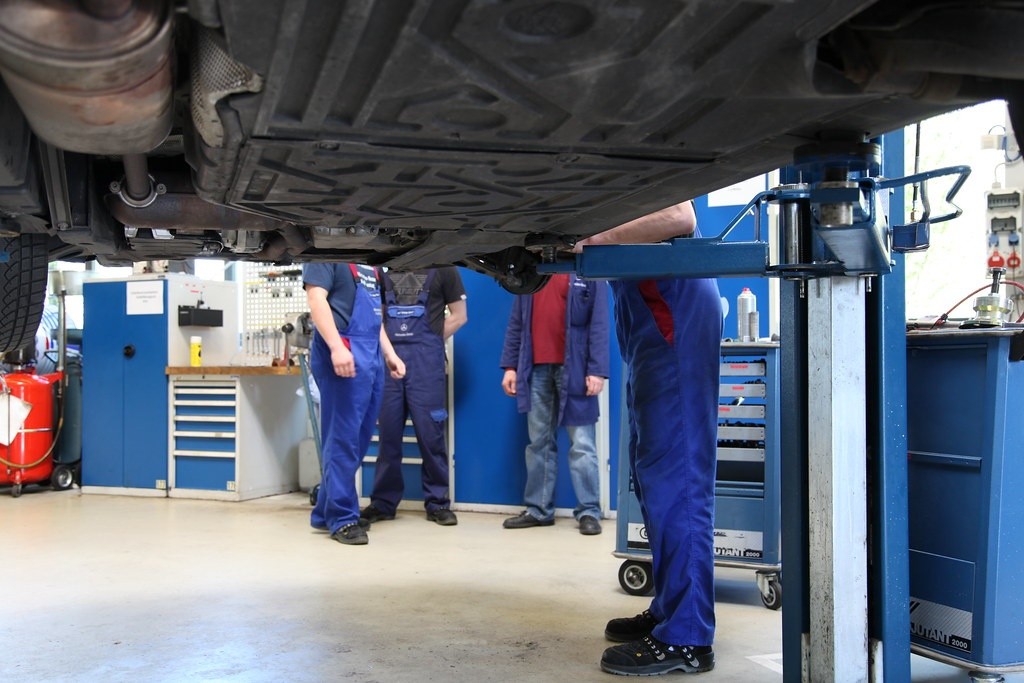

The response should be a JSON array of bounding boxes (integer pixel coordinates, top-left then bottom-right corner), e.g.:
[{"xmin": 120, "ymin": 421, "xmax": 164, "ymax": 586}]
[
  {"xmin": 579, "ymin": 515, "xmax": 603, "ymax": 536},
  {"xmin": 362, "ymin": 503, "xmax": 398, "ymax": 522},
  {"xmin": 426, "ymin": 506, "xmax": 459, "ymax": 526},
  {"xmin": 310, "ymin": 511, "xmax": 372, "ymax": 545},
  {"xmin": 503, "ymin": 510, "xmax": 556, "ymax": 529}
]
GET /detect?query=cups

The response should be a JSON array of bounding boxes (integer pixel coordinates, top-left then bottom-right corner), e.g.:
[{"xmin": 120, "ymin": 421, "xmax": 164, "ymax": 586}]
[{"xmin": 190, "ymin": 336, "xmax": 202, "ymax": 367}]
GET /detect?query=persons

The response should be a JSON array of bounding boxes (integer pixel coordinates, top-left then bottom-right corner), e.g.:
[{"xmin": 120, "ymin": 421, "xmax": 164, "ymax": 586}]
[
  {"xmin": 302, "ymin": 262, "xmax": 406, "ymax": 544},
  {"xmin": 500, "ymin": 273, "xmax": 610, "ymax": 535},
  {"xmin": 360, "ymin": 265, "xmax": 467, "ymax": 525},
  {"xmin": 559, "ymin": 198, "xmax": 723, "ymax": 676}
]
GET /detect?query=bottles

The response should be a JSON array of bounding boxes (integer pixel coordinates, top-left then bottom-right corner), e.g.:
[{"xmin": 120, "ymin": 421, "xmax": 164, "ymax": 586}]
[{"xmin": 736, "ymin": 288, "xmax": 757, "ymax": 339}]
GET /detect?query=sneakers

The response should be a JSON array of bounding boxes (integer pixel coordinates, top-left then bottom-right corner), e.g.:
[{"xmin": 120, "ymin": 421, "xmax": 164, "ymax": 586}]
[{"xmin": 600, "ymin": 607, "xmax": 715, "ymax": 676}]
